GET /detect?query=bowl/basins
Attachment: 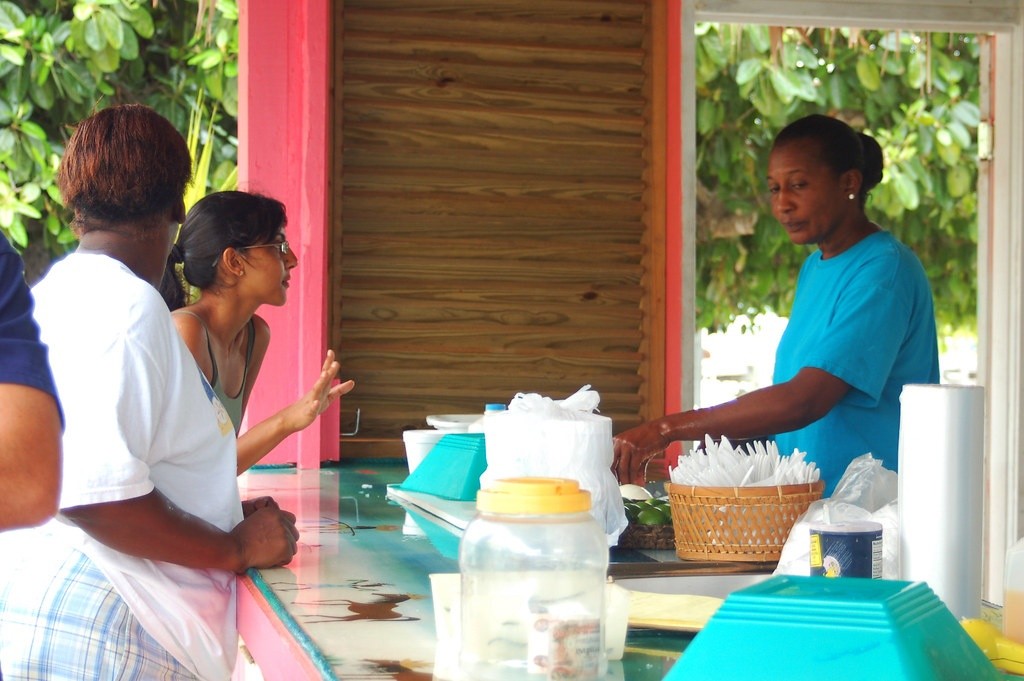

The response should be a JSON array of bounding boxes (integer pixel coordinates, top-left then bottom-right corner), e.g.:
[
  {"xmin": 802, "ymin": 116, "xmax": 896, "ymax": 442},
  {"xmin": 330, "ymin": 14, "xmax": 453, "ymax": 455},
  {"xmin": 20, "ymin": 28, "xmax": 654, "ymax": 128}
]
[{"xmin": 402, "ymin": 429, "xmax": 463, "ymax": 475}]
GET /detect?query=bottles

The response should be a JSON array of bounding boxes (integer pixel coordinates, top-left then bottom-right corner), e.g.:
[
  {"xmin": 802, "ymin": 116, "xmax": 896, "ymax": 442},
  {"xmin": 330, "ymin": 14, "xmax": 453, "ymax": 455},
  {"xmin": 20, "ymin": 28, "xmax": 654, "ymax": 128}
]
[
  {"xmin": 469, "ymin": 403, "xmax": 506, "ymax": 434},
  {"xmin": 458, "ymin": 477, "xmax": 608, "ymax": 681}
]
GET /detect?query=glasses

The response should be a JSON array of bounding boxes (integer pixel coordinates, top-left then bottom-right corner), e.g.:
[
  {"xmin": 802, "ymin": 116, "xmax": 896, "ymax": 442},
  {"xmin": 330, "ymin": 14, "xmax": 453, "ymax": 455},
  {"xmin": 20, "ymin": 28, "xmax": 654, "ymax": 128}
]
[{"xmin": 211, "ymin": 240, "xmax": 288, "ymax": 268}]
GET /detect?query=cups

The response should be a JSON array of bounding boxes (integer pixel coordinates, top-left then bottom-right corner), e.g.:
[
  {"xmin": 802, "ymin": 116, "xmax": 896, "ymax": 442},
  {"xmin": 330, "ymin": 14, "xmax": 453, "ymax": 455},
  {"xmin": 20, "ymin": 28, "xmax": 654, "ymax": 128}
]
[{"xmin": 808, "ymin": 519, "xmax": 883, "ymax": 579}]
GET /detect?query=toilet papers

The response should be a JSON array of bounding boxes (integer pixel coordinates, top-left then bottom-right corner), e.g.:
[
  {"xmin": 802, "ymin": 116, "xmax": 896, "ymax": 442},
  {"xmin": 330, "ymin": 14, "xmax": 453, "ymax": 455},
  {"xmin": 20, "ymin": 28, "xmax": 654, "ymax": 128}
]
[
  {"xmin": 809, "ymin": 521, "xmax": 882, "ymax": 578},
  {"xmin": 896, "ymin": 382, "xmax": 987, "ymax": 624}
]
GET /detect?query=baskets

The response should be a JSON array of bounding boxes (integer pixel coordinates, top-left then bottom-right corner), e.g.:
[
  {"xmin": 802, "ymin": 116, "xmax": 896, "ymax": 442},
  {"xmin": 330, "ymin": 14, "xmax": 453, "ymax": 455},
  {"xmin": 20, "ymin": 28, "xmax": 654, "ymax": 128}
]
[{"xmin": 665, "ymin": 480, "xmax": 825, "ymax": 563}]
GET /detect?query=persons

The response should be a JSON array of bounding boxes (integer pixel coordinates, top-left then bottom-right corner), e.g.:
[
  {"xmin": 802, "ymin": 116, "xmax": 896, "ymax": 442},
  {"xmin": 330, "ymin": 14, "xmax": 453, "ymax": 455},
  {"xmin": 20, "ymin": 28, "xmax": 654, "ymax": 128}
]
[
  {"xmin": 0, "ymin": 103, "xmax": 353, "ymax": 681},
  {"xmin": 611, "ymin": 112, "xmax": 940, "ymax": 499}
]
